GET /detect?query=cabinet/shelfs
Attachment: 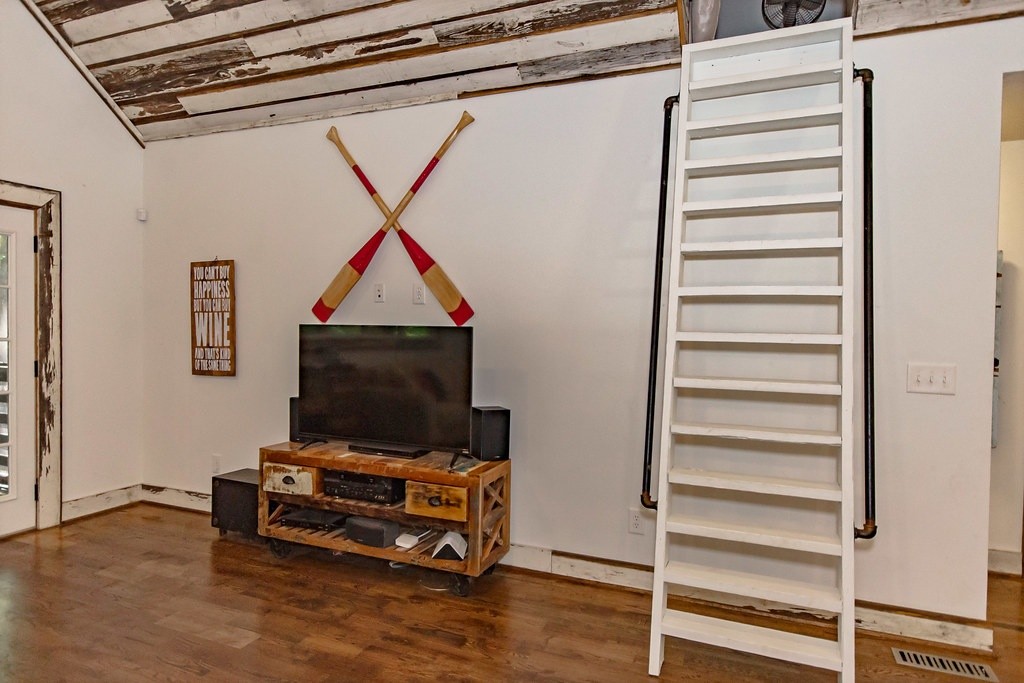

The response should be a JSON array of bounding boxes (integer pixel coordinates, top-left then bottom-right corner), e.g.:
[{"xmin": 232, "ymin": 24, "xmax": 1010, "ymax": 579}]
[{"xmin": 257, "ymin": 440, "xmax": 512, "ymax": 595}]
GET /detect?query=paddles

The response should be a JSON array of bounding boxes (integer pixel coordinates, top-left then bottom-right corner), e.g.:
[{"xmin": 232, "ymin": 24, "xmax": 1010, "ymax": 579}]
[
  {"xmin": 325, "ymin": 123, "xmax": 476, "ymax": 327},
  {"xmin": 310, "ymin": 109, "xmax": 475, "ymax": 323}
]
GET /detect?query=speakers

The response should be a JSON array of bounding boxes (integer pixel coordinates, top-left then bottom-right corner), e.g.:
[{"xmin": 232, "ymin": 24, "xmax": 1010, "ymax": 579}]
[
  {"xmin": 346, "ymin": 517, "xmax": 400, "ymax": 549},
  {"xmin": 471, "ymin": 406, "xmax": 509, "ymax": 461},
  {"xmin": 290, "ymin": 398, "xmax": 327, "ymax": 443}
]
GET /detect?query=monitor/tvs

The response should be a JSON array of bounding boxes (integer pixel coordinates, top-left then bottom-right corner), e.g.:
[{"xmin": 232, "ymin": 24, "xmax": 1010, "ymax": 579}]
[{"xmin": 297, "ymin": 324, "xmax": 473, "ymax": 458}]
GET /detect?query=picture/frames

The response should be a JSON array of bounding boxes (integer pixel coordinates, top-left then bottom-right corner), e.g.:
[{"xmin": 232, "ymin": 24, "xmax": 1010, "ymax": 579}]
[{"xmin": 191, "ymin": 259, "xmax": 235, "ymax": 376}]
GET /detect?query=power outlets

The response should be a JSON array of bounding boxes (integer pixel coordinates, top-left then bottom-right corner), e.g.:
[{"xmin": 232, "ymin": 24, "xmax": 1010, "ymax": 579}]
[{"xmin": 628, "ymin": 507, "xmax": 646, "ymax": 535}]
